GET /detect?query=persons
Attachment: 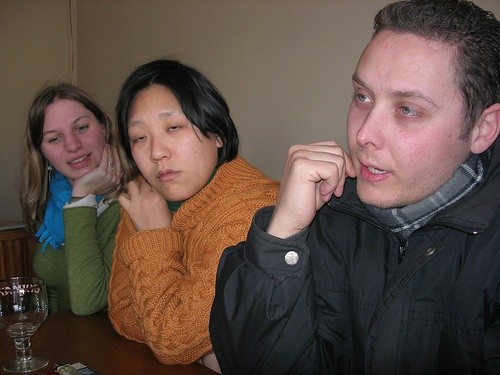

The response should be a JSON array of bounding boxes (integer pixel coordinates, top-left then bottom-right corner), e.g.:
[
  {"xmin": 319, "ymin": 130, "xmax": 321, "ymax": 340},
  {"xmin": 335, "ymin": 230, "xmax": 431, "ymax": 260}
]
[
  {"xmin": 104, "ymin": 60, "xmax": 281, "ymax": 375},
  {"xmin": 209, "ymin": 0, "xmax": 500, "ymax": 375},
  {"xmin": 20, "ymin": 84, "xmax": 126, "ymax": 315}
]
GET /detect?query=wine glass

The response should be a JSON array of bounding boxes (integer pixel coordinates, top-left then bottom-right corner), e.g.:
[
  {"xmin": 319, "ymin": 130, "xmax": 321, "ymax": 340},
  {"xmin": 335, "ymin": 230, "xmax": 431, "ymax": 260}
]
[{"xmin": 0, "ymin": 277, "xmax": 50, "ymax": 373}]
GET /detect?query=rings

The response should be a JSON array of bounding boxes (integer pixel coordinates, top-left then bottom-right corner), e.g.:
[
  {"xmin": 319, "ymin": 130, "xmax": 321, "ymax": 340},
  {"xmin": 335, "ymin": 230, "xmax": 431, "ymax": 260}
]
[{"xmin": 107, "ymin": 162, "xmax": 115, "ymax": 168}]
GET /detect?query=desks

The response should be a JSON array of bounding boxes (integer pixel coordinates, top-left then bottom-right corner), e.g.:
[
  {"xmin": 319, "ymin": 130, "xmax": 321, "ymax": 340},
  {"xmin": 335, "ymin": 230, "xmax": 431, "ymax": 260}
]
[{"xmin": 0, "ymin": 309, "xmax": 221, "ymax": 375}]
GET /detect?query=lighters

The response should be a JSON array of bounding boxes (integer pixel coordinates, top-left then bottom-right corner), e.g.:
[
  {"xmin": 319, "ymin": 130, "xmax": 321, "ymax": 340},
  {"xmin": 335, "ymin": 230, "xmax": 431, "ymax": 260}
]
[{"xmin": 52, "ymin": 359, "xmax": 81, "ymax": 375}]
